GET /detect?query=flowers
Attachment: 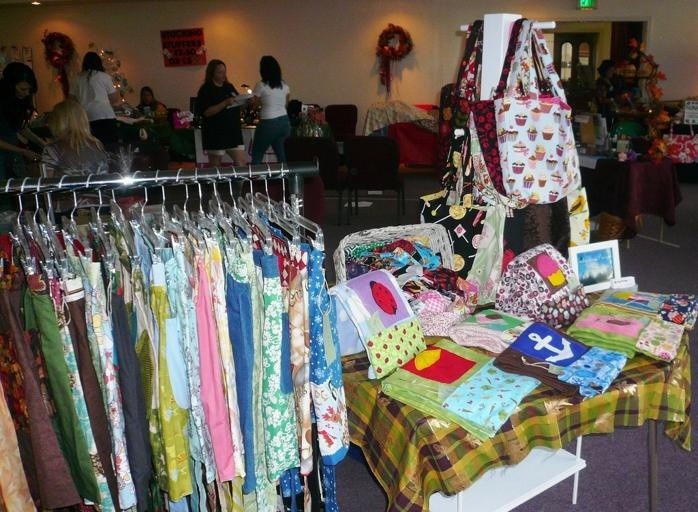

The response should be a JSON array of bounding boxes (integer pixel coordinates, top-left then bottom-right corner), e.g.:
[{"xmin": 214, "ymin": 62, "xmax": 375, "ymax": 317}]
[
  {"xmin": 371, "ymin": 22, "xmax": 414, "ymax": 97},
  {"xmin": 38, "ymin": 28, "xmax": 78, "ymax": 99}
]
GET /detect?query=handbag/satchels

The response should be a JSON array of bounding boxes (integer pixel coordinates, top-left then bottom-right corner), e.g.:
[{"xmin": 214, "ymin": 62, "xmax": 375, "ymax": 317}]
[{"xmin": 416, "ymin": 13, "xmax": 594, "ymax": 330}]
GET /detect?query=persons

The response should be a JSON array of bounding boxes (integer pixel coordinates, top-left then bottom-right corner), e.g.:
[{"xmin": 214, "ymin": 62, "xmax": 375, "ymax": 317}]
[
  {"xmin": 195, "ymin": 58, "xmax": 247, "ymax": 169},
  {"xmin": 0, "ymin": 61, "xmax": 47, "ymax": 179},
  {"xmin": 135, "ymin": 86, "xmax": 168, "ymax": 113},
  {"xmin": 39, "ymin": 98, "xmax": 110, "ymax": 181},
  {"xmin": 251, "ymin": 55, "xmax": 291, "ymax": 165},
  {"xmin": 594, "ymin": 58, "xmax": 616, "ymax": 99},
  {"xmin": 68, "ymin": 51, "xmax": 121, "ymax": 153}
]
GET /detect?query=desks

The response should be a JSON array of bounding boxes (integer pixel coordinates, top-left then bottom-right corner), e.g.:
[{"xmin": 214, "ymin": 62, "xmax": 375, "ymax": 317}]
[
  {"xmin": 660, "ymin": 134, "xmax": 698, "ymax": 169},
  {"xmin": 575, "ymin": 152, "xmax": 682, "ymax": 255},
  {"xmin": 342, "ymin": 287, "xmax": 694, "ymax": 512},
  {"xmin": 192, "ymin": 122, "xmax": 285, "ymax": 169},
  {"xmin": 121, "ymin": 112, "xmax": 170, "ymax": 170}
]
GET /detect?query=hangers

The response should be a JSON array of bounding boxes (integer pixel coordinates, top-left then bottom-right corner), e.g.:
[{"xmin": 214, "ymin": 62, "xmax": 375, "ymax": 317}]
[{"xmin": 1, "ymin": 164, "xmax": 325, "ymax": 292}]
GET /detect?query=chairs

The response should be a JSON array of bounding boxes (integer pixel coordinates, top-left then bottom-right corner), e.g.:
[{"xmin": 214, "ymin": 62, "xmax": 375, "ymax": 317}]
[
  {"xmin": 336, "ymin": 131, "xmax": 405, "ymax": 225},
  {"xmin": 283, "ymin": 133, "xmax": 359, "ymax": 226},
  {"xmin": 324, "ymin": 104, "xmax": 357, "ymax": 136}
]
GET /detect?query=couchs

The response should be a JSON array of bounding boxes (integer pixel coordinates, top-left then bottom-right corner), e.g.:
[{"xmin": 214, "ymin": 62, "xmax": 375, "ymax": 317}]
[{"xmin": 366, "ymin": 99, "xmax": 440, "ymax": 175}]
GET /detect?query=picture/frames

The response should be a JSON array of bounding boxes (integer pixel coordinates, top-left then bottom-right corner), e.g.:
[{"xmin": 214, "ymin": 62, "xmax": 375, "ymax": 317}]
[{"xmin": 567, "ymin": 238, "xmax": 622, "ymax": 294}]
